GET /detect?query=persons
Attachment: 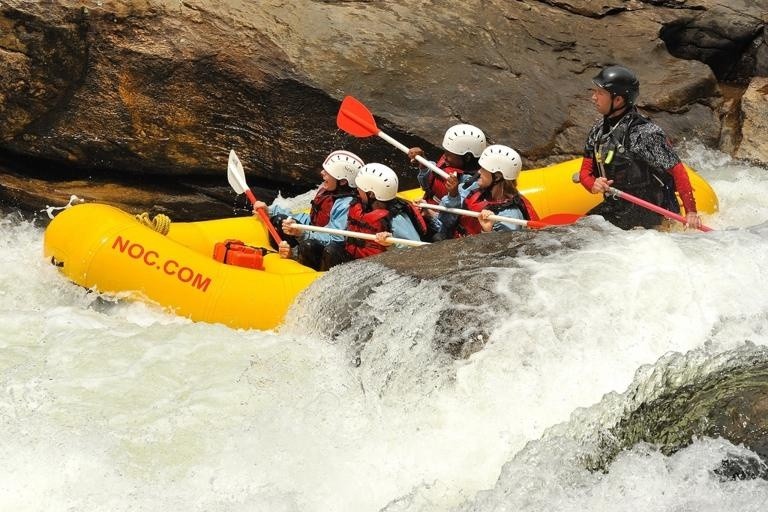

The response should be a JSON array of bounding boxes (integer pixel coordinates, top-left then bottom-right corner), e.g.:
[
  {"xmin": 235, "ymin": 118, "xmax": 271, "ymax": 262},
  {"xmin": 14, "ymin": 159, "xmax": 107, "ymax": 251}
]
[
  {"xmin": 409, "ymin": 123, "xmax": 486, "ymax": 234},
  {"xmin": 252, "ymin": 150, "xmax": 365, "ymax": 259},
  {"xmin": 581, "ymin": 67, "xmax": 702, "ymax": 234},
  {"xmin": 281, "ymin": 161, "xmax": 426, "ymax": 272},
  {"xmin": 431, "ymin": 145, "xmax": 527, "ymax": 239}
]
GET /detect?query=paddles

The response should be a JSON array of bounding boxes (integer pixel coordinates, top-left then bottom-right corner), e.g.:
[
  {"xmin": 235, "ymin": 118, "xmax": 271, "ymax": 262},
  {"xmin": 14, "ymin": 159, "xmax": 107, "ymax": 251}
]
[
  {"xmin": 336, "ymin": 96, "xmax": 458, "ymax": 185},
  {"xmin": 414, "ymin": 200, "xmax": 585, "ymax": 231},
  {"xmin": 227, "ymin": 149, "xmax": 282, "ymax": 245}
]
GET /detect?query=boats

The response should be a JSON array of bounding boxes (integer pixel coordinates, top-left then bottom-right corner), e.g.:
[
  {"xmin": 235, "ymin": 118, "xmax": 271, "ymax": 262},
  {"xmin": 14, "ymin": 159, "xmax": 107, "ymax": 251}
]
[{"xmin": 38, "ymin": 148, "xmax": 725, "ymax": 341}]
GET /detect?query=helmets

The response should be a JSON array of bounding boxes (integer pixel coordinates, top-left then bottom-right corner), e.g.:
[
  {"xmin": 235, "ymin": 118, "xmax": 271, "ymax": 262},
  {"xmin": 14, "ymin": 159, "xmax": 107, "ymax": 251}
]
[
  {"xmin": 593, "ymin": 64, "xmax": 640, "ymax": 98},
  {"xmin": 478, "ymin": 143, "xmax": 523, "ymax": 182},
  {"xmin": 442, "ymin": 123, "xmax": 488, "ymax": 159},
  {"xmin": 322, "ymin": 150, "xmax": 365, "ymax": 189},
  {"xmin": 354, "ymin": 162, "xmax": 400, "ymax": 201}
]
